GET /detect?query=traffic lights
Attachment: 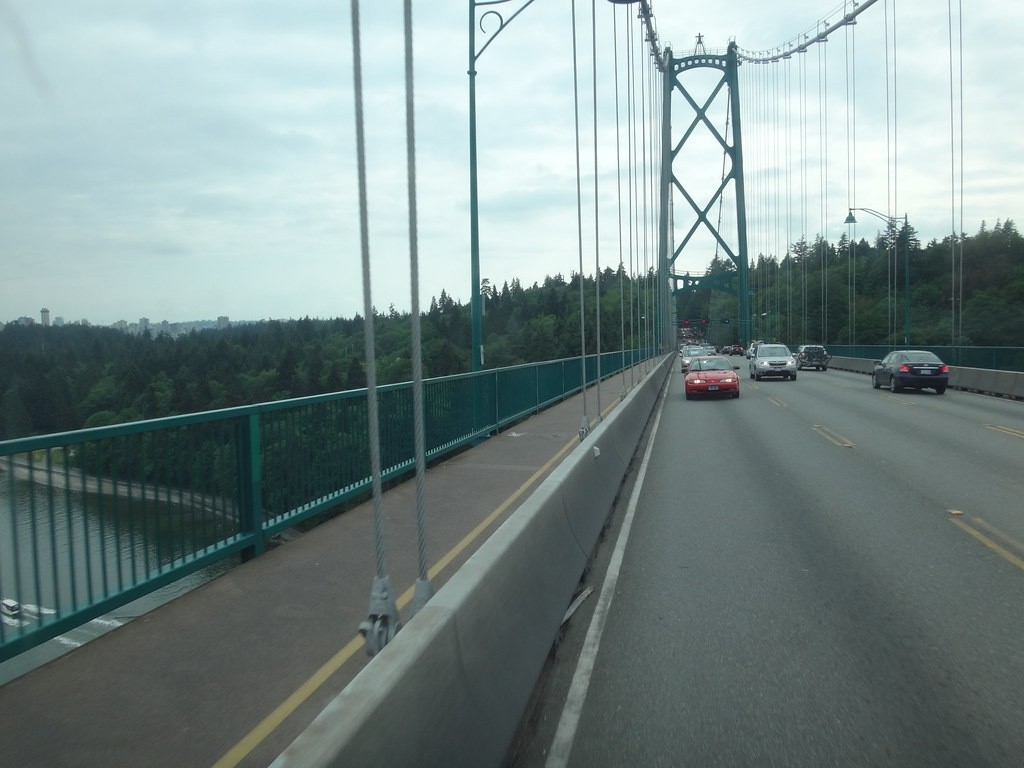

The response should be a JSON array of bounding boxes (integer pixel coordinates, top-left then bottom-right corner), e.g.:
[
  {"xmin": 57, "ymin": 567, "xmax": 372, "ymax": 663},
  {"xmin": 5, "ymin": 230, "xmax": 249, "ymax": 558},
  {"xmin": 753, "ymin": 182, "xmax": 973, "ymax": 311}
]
[
  {"xmin": 680, "ymin": 319, "xmax": 689, "ymax": 324},
  {"xmin": 700, "ymin": 318, "xmax": 710, "ymax": 324},
  {"xmin": 721, "ymin": 318, "xmax": 729, "ymax": 323}
]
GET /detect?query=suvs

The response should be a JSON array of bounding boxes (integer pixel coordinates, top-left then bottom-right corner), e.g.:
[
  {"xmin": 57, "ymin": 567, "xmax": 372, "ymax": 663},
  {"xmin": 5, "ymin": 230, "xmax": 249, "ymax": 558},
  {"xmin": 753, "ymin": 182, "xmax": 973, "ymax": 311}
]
[
  {"xmin": 728, "ymin": 345, "xmax": 744, "ymax": 356},
  {"xmin": 746, "ymin": 341, "xmax": 798, "ymax": 382},
  {"xmin": 746, "ymin": 341, "xmax": 765, "ymax": 360},
  {"xmin": 722, "ymin": 346, "xmax": 731, "ymax": 354},
  {"xmin": 795, "ymin": 344, "xmax": 829, "ymax": 371}
]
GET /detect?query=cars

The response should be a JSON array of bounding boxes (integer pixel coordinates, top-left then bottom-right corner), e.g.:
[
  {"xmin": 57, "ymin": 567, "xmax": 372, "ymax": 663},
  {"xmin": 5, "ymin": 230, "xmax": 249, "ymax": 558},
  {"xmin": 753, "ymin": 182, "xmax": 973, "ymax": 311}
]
[
  {"xmin": 681, "ymin": 356, "xmax": 741, "ymax": 399},
  {"xmin": 871, "ymin": 350, "xmax": 950, "ymax": 394},
  {"xmin": 678, "ymin": 327, "xmax": 720, "ymax": 373}
]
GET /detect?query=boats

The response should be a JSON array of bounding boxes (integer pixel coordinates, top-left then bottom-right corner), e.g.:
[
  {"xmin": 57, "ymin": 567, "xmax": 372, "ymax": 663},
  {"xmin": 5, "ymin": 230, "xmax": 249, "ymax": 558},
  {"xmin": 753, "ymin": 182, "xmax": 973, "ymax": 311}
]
[{"xmin": 1, "ymin": 597, "xmax": 25, "ymax": 616}]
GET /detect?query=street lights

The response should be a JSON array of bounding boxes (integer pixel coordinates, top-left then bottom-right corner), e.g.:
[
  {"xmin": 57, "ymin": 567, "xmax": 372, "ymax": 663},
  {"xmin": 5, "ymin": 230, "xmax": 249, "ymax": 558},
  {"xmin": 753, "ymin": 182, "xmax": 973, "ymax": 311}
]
[
  {"xmin": 843, "ymin": 207, "xmax": 911, "ymax": 349},
  {"xmin": 464, "ymin": 0, "xmax": 644, "ymax": 445}
]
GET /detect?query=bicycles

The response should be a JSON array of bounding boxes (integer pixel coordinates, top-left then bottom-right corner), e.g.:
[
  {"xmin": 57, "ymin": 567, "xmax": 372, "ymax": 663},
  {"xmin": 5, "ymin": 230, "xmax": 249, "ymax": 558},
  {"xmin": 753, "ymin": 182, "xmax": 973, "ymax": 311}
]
[{"xmin": 796, "ymin": 346, "xmax": 833, "ymax": 369}]
